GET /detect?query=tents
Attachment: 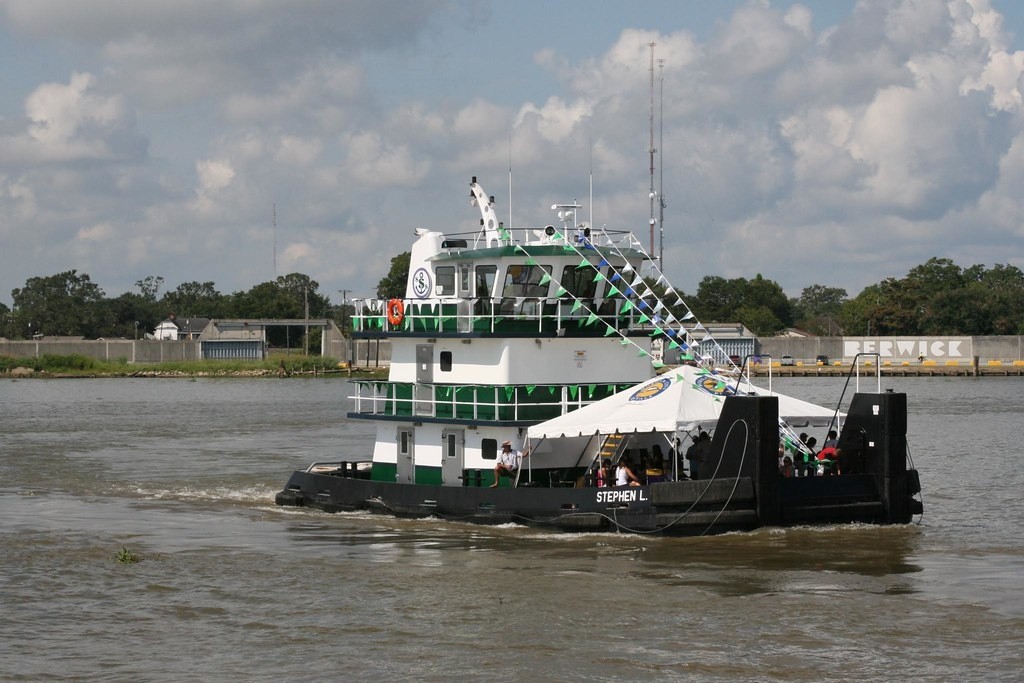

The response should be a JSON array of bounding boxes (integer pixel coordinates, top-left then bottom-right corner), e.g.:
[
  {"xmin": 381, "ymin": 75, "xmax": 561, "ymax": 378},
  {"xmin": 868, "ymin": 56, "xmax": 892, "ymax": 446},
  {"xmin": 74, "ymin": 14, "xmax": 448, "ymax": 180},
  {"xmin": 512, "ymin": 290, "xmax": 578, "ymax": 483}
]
[{"xmin": 528, "ymin": 364, "xmax": 849, "ymax": 486}]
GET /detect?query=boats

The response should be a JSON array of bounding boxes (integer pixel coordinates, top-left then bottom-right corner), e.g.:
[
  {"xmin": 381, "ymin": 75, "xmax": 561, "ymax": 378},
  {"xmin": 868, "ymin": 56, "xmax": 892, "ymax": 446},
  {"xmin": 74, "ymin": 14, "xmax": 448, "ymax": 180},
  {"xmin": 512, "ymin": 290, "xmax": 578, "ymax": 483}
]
[{"xmin": 274, "ymin": 176, "xmax": 926, "ymax": 541}]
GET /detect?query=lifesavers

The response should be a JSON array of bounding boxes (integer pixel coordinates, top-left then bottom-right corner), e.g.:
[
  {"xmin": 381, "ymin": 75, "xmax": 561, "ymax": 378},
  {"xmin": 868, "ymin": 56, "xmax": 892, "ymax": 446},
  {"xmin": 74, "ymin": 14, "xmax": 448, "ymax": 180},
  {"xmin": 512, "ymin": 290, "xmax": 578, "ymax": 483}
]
[
  {"xmin": 387, "ymin": 299, "xmax": 404, "ymax": 325},
  {"xmin": 817, "ymin": 446, "xmax": 836, "ymax": 460}
]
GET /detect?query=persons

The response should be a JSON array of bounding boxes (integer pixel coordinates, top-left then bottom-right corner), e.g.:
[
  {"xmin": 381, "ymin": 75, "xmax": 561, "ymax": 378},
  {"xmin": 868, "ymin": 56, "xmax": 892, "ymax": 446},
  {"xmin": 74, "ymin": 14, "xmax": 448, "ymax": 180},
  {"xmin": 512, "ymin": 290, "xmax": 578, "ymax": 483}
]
[
  {"xmin": 780, "ymin": 431, "xmax": 840, "ymax": 476},
  {"xmin": 598, "ymin": 431, "xmax": 712, "ymax": 487},
  {"xmin": 489, "ymin": 440, "xmax": 531, "ymax": 488}
]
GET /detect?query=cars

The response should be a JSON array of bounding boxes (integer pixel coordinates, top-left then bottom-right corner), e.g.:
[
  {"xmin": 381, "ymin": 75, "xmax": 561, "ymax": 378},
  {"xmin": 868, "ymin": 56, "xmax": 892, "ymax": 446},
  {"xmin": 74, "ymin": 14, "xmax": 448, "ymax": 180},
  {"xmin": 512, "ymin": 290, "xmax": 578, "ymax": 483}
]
[
  {"xmin": 816, "ymin": 354, "xmax": 830, "ymax": 365},
  {"xmin": 779, "ymin": 354, "xmax": 793, "ymax": 366},
  {"xmin": 727, "ymin": 354, "xmax": 743, "ymax": 367}
]
